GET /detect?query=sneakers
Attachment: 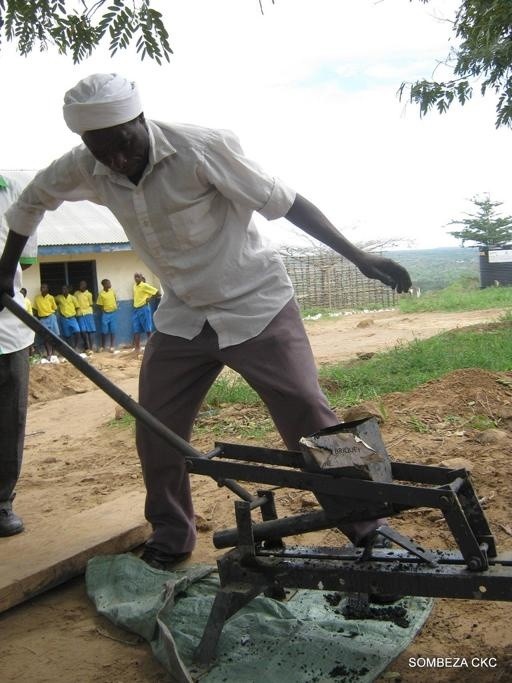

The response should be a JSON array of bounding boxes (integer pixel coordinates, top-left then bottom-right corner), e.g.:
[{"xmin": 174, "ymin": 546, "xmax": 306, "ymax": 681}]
[
  {"xmin": 139, "ymin": 544, "xmax": 192, "ymax": 573},
  {"xmin": 360, "ymin": 536, "xmax": 402, "ymax": 604},
  {"xmin": 0, "ymin": 509, "xmax": 24, "ymax": 537}
]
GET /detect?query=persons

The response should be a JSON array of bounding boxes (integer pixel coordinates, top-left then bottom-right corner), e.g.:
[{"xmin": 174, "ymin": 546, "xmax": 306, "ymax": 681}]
[
  {"xmin": 130, "ymin": 272, "xmax": 162, "ymax": 354},
  {"xmin": 96, "ymin": 279, "xmax": 118, "ymax": 353},
  {"xmin": 32, "ymin": 283, "xmax": 60, "ymax": 360},
  {"xmin": 20, "ymin": 287, "xmax": 33, "ymax": 317},
  {"xmin": 1, "ymin": 176, "xmax": 38, "ymax": 537},
  {"xmin": 73, "ymin": 280, "xmax": 97, "ymax": 351},
  {"xmin": 1, "ymin": 71, "xmax": 413, "ymax": 606},
  {"xmin": 53, "ymin": 284, "xmax": 81, "ymax": 349}
]
